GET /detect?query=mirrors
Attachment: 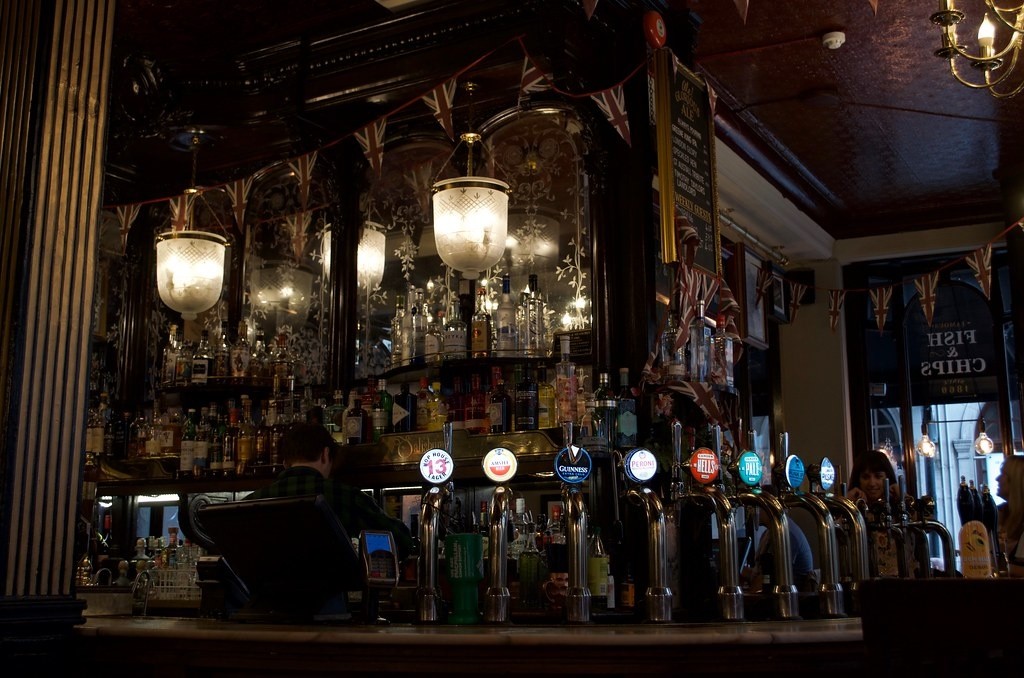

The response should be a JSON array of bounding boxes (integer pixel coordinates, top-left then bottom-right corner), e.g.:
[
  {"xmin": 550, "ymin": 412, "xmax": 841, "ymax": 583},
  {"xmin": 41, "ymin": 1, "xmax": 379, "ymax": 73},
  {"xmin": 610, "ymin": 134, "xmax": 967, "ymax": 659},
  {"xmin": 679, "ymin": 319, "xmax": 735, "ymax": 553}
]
[{"xmin": 146, "ymin": 199, "xmax": 329, "ymax": 391}]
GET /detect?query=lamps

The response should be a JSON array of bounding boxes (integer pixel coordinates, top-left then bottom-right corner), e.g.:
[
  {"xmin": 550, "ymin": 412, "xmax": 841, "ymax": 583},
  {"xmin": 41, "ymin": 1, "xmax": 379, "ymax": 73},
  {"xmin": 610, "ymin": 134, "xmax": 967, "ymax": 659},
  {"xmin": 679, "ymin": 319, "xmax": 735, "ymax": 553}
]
[
  {"xmin": 154, "ymin": 130, "xmax": 227, "ymax": 322},
  {"xmin": 929, "ymin": 0, "xmax": 1024, "ymax": 100},
  {"xmin": 429, "ymin": 81, "xmax": 510, "ymax": 282},
  {"xmin": 322, "ymin": 218, "xmax": 386, "ymax": 296},
  {"xmin": 251, "ymin": 262, "xmax": 313, "ymax": 331}
]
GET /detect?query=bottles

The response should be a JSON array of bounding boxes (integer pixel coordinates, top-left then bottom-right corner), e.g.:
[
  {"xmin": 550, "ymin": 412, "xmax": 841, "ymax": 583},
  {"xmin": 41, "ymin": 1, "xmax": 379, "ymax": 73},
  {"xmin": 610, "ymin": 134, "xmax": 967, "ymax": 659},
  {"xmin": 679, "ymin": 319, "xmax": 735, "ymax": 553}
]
[{"xmin": 78, "ymin": 276, "xmax": 734, "ymax": 623}]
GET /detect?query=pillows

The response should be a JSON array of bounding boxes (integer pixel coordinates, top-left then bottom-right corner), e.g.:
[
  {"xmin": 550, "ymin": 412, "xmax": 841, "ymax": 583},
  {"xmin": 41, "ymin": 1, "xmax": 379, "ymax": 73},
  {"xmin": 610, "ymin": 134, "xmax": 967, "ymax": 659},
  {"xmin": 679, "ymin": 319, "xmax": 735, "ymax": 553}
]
[{"xmin": 354, "ymin": 108, "xmax": 591, "ymax": 382}]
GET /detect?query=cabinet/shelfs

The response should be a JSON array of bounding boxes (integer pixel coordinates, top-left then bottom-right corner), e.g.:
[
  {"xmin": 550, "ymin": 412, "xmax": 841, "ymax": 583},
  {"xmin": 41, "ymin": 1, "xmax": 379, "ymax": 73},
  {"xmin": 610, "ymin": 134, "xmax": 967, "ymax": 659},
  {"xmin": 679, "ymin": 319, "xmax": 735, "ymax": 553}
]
[{"xmin": 101, "ymin": 350, "xmax": 599, "ymax": 584}]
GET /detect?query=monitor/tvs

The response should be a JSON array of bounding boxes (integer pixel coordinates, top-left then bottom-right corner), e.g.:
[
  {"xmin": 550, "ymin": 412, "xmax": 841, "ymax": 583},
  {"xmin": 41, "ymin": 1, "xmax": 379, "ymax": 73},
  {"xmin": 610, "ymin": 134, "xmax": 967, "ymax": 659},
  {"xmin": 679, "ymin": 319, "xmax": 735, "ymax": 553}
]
[{"xmin": 198, "ymin": 500, "xmax": 367, "ymax": 617}]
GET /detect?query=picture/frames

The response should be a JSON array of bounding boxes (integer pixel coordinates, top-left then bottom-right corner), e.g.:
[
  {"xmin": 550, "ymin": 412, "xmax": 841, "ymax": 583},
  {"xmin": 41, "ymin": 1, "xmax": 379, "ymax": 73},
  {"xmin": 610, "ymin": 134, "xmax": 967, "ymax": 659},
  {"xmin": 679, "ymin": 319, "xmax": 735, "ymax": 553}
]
[{"xmin": 706, "ymin": 234, "xmax": 790, "ymax": 351}]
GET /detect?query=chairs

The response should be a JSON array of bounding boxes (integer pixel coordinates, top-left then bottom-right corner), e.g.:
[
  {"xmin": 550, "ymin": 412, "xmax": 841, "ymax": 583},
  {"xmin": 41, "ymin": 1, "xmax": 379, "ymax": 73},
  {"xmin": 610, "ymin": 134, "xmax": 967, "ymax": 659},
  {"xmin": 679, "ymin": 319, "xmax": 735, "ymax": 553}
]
[{"xmin": 859, "ymin": 577, "xmax": 1024, "ymax": 678}]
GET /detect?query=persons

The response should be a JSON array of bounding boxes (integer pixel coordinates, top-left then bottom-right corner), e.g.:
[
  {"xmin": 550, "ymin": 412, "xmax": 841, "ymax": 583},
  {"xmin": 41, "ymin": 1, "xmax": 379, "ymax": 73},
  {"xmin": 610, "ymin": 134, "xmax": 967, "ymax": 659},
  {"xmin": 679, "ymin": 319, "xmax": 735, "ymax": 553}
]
[
  {"xmin": 757, "ymin": 485, "xmax": 813, "ymax": 587},
  {"xmin": 847, "ymin": 451, "xmax": 933, "ymax": 580},
  {"xmin": 240, "ymin": 422, "xmax": 413, "ymax": 564},
  {"xmin": 995, "ymin": 455, "xmax": 1024, "ymax": 575}
]
